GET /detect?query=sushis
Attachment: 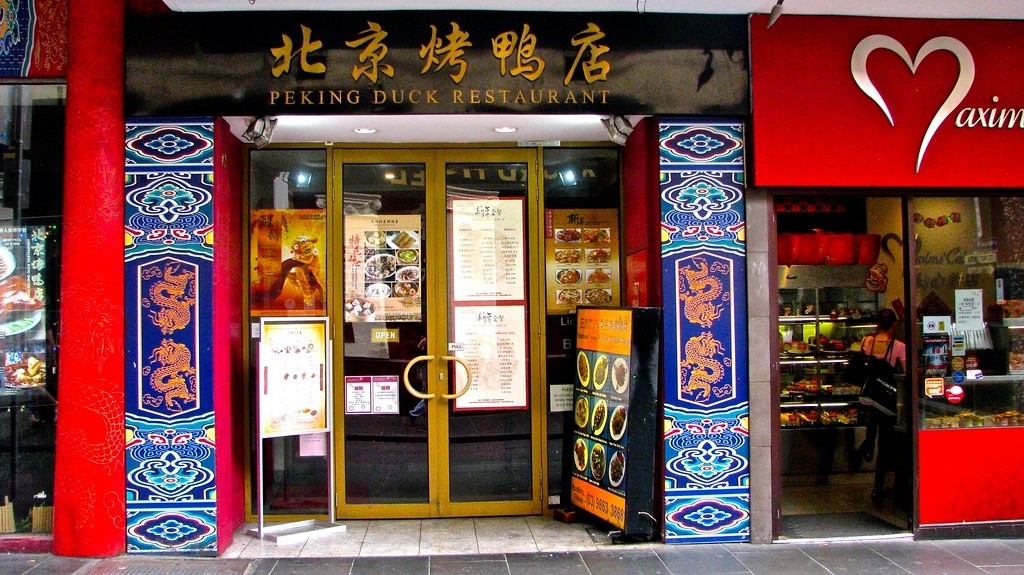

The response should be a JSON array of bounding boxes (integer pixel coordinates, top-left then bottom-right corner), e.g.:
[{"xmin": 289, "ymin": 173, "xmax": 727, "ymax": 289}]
[{"xmin": 399, "ymin": 283, "xmax": 415, "ymax": 296}]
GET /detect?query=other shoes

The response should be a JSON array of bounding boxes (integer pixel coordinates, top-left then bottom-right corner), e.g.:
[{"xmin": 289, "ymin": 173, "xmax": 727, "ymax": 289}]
[
  {"xmin": 871, "ymin": 483, "xmax": 884, "ymax": 502},
  {"xmin": 863, "ymin": 439, "xmax": 874, "ymax": 461},
  {"xmin": 409, "ymin": 413, "xmax": 417, "ymax": 427}
]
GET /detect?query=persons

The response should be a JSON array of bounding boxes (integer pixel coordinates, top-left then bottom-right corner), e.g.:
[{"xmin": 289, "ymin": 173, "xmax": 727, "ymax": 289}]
[{"xmin": 859, "ymin": 308, "xmax": 906, "ymax": 502}]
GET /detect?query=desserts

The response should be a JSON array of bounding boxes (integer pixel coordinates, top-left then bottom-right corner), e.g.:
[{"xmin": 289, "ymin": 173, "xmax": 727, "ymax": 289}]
[{"xmin": 782, "ymin": 305, "xmax": 871, "ymax": 424}]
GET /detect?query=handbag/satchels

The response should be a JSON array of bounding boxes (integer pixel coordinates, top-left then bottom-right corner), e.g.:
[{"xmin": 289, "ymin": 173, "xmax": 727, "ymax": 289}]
[
  {"xmin": 842, "ymin": 335, "xmax": 876, "ymax": 389},
  {"xmin": 858, "ymin": 338, "xmax": 898, "ymax": 417}
]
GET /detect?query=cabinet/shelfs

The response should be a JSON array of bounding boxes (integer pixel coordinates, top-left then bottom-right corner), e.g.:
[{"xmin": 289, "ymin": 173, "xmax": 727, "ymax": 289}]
[{"xmin": 778, "ymin": 264, "xmax": 880, "ymax": 477}]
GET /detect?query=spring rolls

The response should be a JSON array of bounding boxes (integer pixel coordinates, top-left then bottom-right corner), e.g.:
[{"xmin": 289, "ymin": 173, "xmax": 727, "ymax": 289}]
[{"xmin": 393, "ymin": 232, "xmax": 415, "ymax": 248}]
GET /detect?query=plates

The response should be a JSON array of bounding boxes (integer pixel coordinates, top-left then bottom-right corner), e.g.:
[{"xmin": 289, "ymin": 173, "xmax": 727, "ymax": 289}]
[
  {"xmin": 0, "ymin": 242, "xmax": 47, "ymax": 390},
  {"xmin": 591, "ymin": 399, "xmax": 607, "ymax": 436},
  {"xmin": 363, "ymin": 253, "xmax": 395, "ymax": 279},
  {"xmin": 557, "ymin": 289, "xmax": 582, "ymax": 303},
  {"xmin": 608, "ymin": 450, "xmax": 627, "ymax": 487},
  {"xmin": 395, "ymin": 266, "xmax": 420, "ymax": 282},
  {"xmin": 586, "ymin": 268, "xmax": 611, "ymax": 283},
  {"xmin": 585, "ymin": 289, "xmax": 612, "ymax": 304},
  {"xmin": 365, "ymin": 283, "xmax": 391, "ymax": 298},
  {"xmin": 591, "ymin": 443, "xmax": 605, "ymax": 481},
  {"xmin": 555, "ymin": 250, "xmax": 581, "ymax": 263},
  {"xmin": 609, "ymin": 404, "xmax": 627, "ymax": 442},
  {"xmin": 555, "ymin": 231, "xmax": 579, "ymax": 242},
  {"xmin": 556, "ymin": 268, "xmax": 582, "ymax": 284},
  {"xmin": 592, "ymin": 354, "xmax": 609, "ymax": 390},
  {"xmin": 572, "ymin": 437, "xmax": 587, "ymax": 471},
  {"xmin": 574, "ymin": 395, "xmax": 590, "ymax": 428},
  {"xmin": 577, "ymin": 351, "xmax": 591, "ymax": 387},
  {"xmin": 387, "ymin": 230, "xmax": 418, "ymax": 249},
  {"xmin": 612, "ymin": 358, "xmax": 629, "ymax": 394}
]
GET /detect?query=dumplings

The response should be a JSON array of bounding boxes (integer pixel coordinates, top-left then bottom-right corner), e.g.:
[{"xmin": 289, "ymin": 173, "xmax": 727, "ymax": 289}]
[{"xmin": 344, "ymin": 299, "xmax": 371, "ymax": 316}]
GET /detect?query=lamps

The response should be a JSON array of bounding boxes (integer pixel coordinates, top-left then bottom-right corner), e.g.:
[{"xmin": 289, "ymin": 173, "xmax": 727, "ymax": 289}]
[
  {"xmin": 287, "ymin": 168, "xmax": 312, "ymax": 189},
  {"xmin": 600, "ymin": 116, "xmax": 633, "ymax": 146},
  {"xmin": 557, "ymin": 164, "xmax": 584, "ymax": 189},
  {"xmin": 242, "ymin": 118, "xmax": 278, "ymax": 150}
]
